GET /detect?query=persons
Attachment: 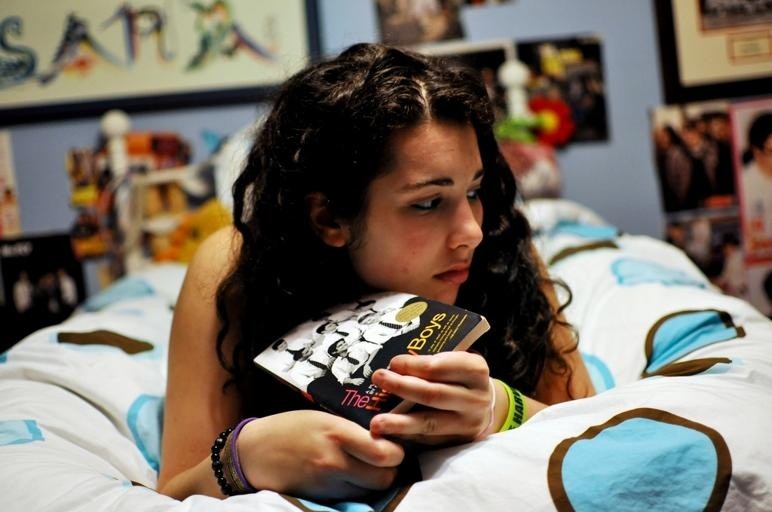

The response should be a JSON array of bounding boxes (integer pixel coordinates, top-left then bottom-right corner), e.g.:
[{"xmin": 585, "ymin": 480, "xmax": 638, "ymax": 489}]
[
  {"xmin": 268, "ymin": 296, "xmax": 435, "ymax": 403},
  {"xmin": 649, "ymin": 110, "xmax": 772, "ymax": 287},
  {"xmin": 157, "ymin": 41, "xmax": 599, "ymax": 501}
]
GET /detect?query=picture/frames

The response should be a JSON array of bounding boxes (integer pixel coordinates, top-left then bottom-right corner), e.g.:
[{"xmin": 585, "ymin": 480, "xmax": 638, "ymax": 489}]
[
  {"xmin": 0, "ymin": 1, "xmax": 326, "ymax": 131},
  {"xmin": 652, "ymin": 2, "xmax": 772, "ymax": 102},
  {"xmin": 410, "ymin": 39, "xmax": 519, "ymax": 115}
]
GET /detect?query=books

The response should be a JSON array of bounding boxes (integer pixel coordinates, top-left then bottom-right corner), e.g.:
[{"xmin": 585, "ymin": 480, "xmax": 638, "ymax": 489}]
[{"xmin": 250, "ymin": 285, "xmax": 493, "ymax": 429}]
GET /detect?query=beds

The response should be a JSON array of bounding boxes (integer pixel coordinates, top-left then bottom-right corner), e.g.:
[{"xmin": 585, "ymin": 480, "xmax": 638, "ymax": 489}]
[{"xmin": 0, "ymin": 194, "xmax": 770, "ymax": 511}]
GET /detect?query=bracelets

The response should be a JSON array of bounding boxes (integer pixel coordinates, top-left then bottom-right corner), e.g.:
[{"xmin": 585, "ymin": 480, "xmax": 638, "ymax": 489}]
[
  {"xmin": 491, "ymin": 374, "xmax": 526, "ymax": 441},
  {"xmin": 210, "ymin": 416, "xmax": 258, "ymax": 501}
]
